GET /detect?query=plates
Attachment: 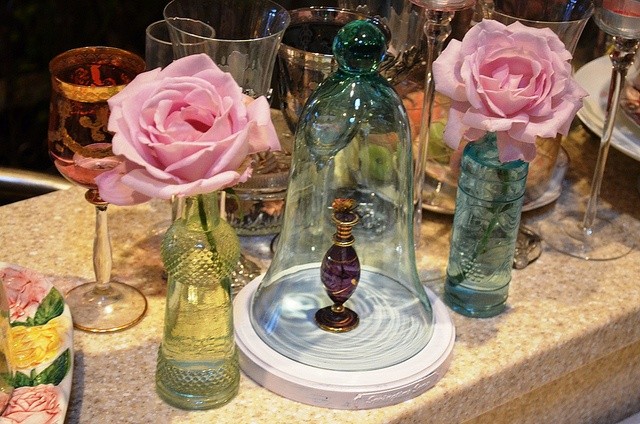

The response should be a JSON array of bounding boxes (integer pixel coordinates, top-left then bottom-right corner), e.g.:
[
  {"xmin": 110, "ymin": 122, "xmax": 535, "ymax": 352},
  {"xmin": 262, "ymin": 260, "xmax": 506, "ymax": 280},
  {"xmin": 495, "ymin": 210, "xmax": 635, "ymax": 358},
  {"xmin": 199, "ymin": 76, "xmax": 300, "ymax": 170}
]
[
  {"xmin": 0, "ymin": 260, "xmax": 75, "ymax": 424},
  {"xmin": 572, "ymin": 53, "xmax": 640, "ymax": 161}
]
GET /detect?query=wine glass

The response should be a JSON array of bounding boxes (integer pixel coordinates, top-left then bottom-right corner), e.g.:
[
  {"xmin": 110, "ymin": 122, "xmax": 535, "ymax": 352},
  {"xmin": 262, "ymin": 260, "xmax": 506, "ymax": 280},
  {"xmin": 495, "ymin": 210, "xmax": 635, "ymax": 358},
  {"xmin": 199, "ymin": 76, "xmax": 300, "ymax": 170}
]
[
  {"xmin": 163, "ymin": 0, "xmax": 291, "ymax": 100},
  {"xmin": 377, "ymin": 2, "xmax": 477, "ymax": 283},
  {"xmin": 538, "ymin": 0, "xmax": 640, "ymax": 262},
  {"xmin": 145, "ymin": 17, "xmax": 216, "ymax": 69},
  {"xmin": 266, "ymin": 3, "xmax": 402, "ymax": 258},
  {"xmin": 381, "ymin": 0, "xmax": 426, "ymax": 89},
  {"xmin": 48, "ymin": 45, "xmax": 148, "ymax": 334}
]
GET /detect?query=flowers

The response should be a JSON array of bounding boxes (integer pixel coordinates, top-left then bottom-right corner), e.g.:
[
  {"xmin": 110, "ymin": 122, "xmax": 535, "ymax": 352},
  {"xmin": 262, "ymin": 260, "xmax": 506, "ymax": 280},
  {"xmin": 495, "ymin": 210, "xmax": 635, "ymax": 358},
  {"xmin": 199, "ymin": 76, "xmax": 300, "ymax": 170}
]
[
  {"xmin": 0, "ymin": 266, "xmax": 49, "ymax": 324},
  {"xmin": 92, "ymin": 53, "xmax": 283, "ymax": 301},
  {"xmin": 0, "ymin": 347, "xmax": 72, "ymax": 424},
  {"xmin": 432, "ymin": 18, "xmax": 591, "ymax": 165},
  {"xmin": 9, "ymin": 286, "xmax": 65, "ymax": 371}
]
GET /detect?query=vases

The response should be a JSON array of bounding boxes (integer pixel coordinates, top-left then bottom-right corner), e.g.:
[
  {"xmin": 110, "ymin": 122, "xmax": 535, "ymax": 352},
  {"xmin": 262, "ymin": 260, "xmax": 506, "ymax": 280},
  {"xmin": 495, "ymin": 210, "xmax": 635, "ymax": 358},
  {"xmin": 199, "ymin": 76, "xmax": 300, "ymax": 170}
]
[
  {"xmin": 443, "ymin": 130, "xmax": 528, "ymax": 319},
  {"xmin": 155, "ymin": 189, "xmax": 240, "ymax": 412}
]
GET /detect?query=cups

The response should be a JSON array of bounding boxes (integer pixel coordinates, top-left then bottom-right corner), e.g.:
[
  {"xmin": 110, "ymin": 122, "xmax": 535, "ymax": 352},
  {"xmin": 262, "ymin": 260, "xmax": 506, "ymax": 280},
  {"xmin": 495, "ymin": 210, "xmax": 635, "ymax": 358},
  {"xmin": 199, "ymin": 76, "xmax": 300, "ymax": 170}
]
[{"xmin": 480, "ymin": 0, "xmax": 597, "ymax": 203}]
